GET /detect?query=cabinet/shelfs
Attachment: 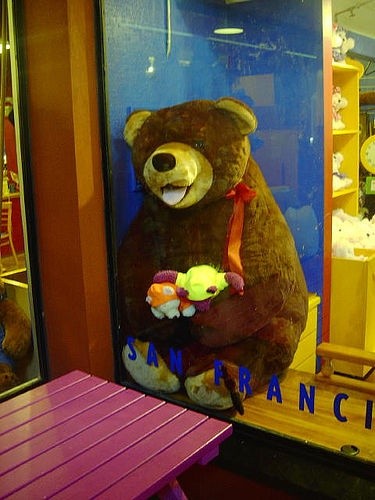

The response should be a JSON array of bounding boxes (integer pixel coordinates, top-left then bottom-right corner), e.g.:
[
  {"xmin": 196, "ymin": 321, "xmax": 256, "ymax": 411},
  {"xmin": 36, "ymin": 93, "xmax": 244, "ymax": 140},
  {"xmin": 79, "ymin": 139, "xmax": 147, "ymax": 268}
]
[
  {"xmin": 331, "ymin": 64, "xmax": 375, "ymax": 377},
  {"xmin": 288, "ymin": 293, "xmax": 321, "ymax": 376}
]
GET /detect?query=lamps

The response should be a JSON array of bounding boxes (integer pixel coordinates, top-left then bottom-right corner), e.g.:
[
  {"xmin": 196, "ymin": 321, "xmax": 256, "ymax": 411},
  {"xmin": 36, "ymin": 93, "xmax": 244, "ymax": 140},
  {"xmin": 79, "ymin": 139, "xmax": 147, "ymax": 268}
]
[{"xmin": 213, "ymin": 14, "xmax": 245, "ymax": 37}]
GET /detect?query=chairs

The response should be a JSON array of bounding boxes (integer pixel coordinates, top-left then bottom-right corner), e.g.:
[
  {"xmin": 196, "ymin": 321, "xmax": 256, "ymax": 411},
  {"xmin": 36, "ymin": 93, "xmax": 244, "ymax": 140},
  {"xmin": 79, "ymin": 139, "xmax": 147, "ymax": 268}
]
[{"xmin": 0, "ymin": 201, "xmax": 19, "ymax": 267}]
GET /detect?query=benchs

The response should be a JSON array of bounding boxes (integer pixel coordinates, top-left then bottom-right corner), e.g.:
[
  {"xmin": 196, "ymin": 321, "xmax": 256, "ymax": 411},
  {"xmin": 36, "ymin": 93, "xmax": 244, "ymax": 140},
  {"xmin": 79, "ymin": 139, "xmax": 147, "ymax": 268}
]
[{"xmin": 0, "ymin": 367, "xmax": 233, "ymax": 500}]
